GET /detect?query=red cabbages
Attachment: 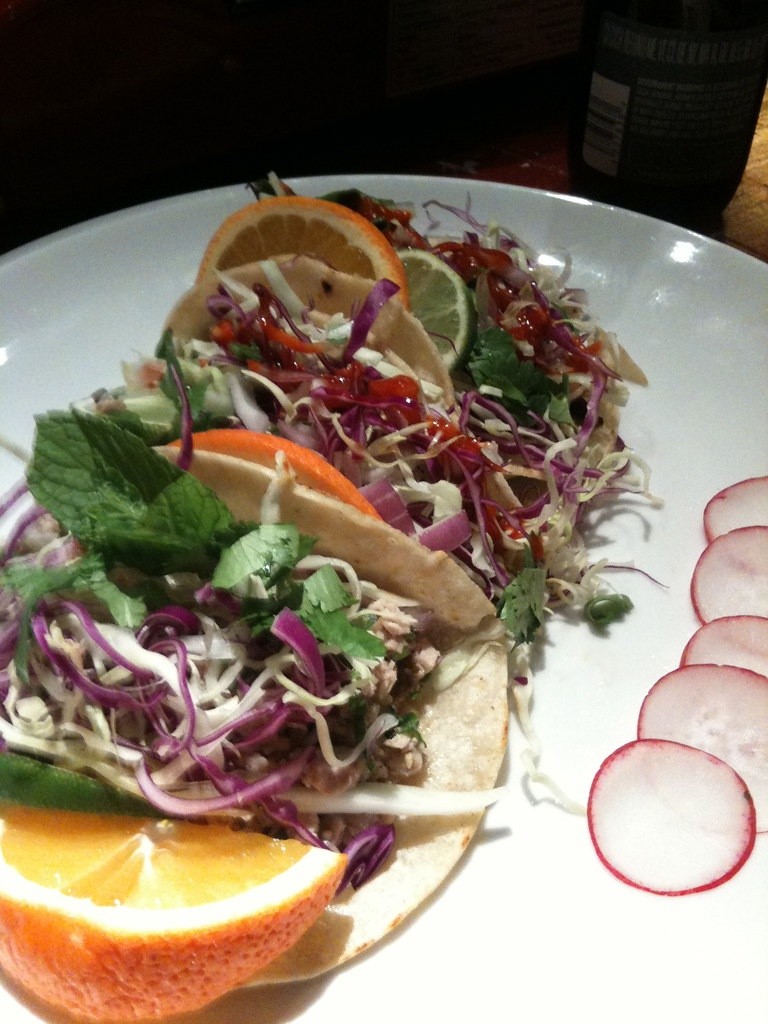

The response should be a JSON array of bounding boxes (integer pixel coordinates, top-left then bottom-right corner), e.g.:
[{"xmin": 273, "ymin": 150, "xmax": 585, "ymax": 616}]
[{"xmin": 1, "ymin": 190, "xmax": 666, "ymax": 888}]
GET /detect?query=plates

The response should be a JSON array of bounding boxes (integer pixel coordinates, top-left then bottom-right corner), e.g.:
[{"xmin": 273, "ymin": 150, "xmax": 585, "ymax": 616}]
[{"xmin": 1, "ymin": 171, "xmax": 768, "ymax": 1017}]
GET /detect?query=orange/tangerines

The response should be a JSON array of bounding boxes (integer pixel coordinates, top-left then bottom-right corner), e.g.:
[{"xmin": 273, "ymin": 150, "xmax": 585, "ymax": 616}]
[{"xmin": 0, "ymin": 801, "xmax": 349, "ymax": 1022}]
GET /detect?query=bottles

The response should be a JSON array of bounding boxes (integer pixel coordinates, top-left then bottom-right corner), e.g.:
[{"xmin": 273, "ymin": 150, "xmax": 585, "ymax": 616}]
[{"xmin": 569, "ymin": 0, "xmax": 768, "ymax": 215}]
